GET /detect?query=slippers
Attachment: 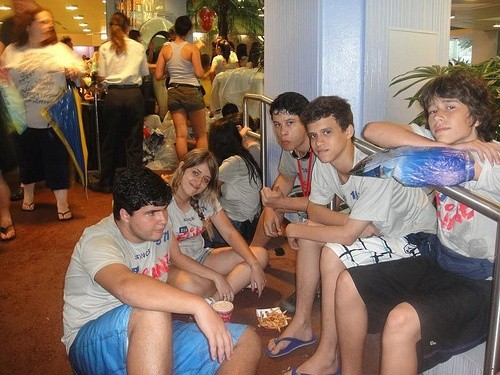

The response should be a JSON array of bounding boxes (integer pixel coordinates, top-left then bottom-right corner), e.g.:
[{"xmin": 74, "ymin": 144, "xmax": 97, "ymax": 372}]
[
  {"xmin": 57, "ymin": 205, "xmax": 72, "ymax": 221},
  {"xmin": 0, "ymin": 224, "xmax": 17, "ymax": 241},
  {"xmin": 21, "ymin": 200, "xmax": 35, "ymax": 212},
  {"xmin": 280, "ymin": 360, "xmax": 342, "ymax": 375},
  {"xmin": 264, "ymin": 335, "xmax": 318, "ymax": 358}
]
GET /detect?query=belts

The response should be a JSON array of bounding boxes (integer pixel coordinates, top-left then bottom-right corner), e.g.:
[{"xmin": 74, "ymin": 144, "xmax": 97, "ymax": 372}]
[
  {"xmin": 107, "ymin": 83, "xmax": 140, "ymax": 90},
  {"xmin": 169, "ymin": 82, "xmax": 198, "ymax": 88}
]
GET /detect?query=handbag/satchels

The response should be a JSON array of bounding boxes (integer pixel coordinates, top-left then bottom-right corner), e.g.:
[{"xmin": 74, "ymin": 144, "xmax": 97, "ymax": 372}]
[{"xmin": 197, "ymin": 85, "xmax": 206, "ymax": 96}]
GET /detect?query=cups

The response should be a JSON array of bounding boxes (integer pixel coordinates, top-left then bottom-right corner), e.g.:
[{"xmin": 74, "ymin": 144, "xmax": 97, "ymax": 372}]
[{"xmin": 212, "ymin": 301, "xmax": 235, "ymax": 323}]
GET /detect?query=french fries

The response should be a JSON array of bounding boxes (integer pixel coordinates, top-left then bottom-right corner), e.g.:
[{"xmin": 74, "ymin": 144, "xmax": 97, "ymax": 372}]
[{"xmin": 258, "ymin": 310, "xmax": 292, "ymax": 333}]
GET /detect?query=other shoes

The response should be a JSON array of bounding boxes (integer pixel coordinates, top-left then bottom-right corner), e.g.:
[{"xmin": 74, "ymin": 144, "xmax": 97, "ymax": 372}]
[
  {"xmin": 10, "ymin": 186, "xmax": 24, "ymax": 202},
  {"xmin": 103, "ymin": 181, "xmax": 113, "ymax": 192}
]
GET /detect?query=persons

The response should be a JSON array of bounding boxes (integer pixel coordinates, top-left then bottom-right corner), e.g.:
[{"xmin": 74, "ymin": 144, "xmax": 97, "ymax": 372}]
[
  {"xmin": 248, "ymin": 91, "xmax": 322, "ymax": 313},
  {"xmin": 163, "ymin": 148, "xmax": 269, "ymax": 301},
  {"xmin": 334, "ymin": 75, "xmax": 500, "ymax": 375},
  {"xmin": 1, "ymin": 0, "xmax": 265, "ymax": 240},
  {"xmin": 203, "ymin": 117, "xmax": 263, "ymax": 247},
  {"xmin": 267, "ymin": 94, "xmax": 437, "ymax": 375},
  {"xmin": 62, "ymin": 162, "xmax": 263, "ymax": 375}
]
